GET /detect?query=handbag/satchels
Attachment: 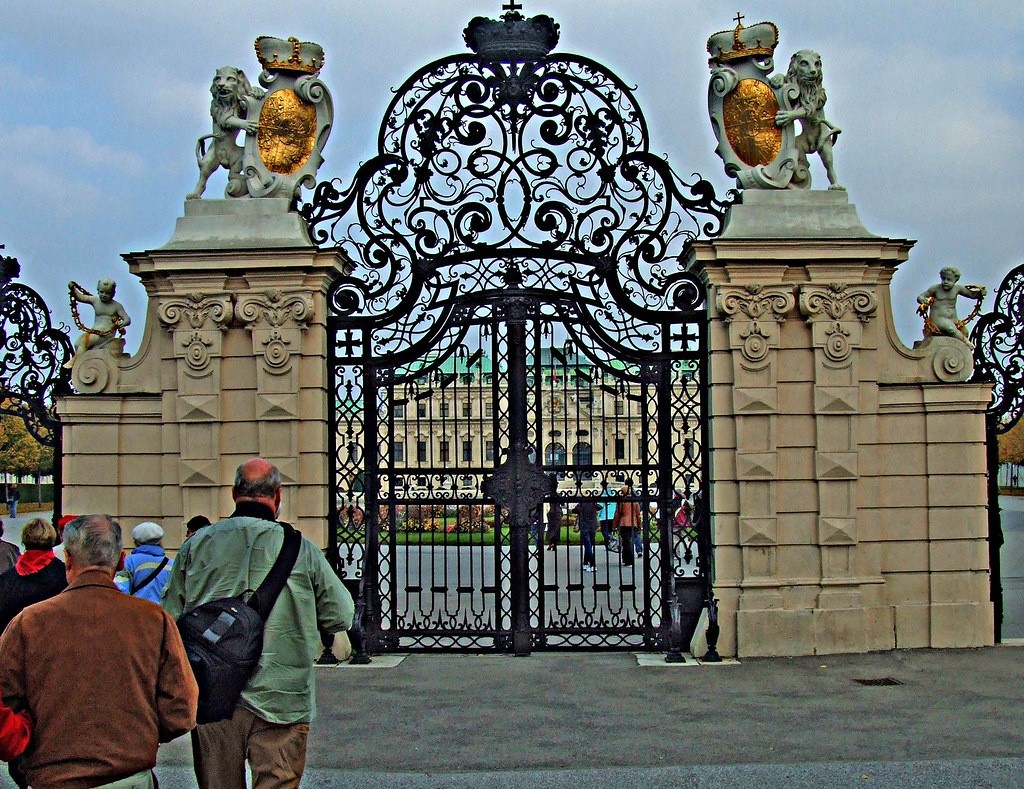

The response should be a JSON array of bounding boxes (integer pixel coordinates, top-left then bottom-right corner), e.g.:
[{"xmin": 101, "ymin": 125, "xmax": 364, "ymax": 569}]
[
  {"xmin": 175, "ymin": 521, "xmax": 302, "ymax": 724},
  {"xmin": 608, "ymin": 530, "xmax": 622, "ymax": 553},
  {"xmin": 7, "ymin": 496, "xmax": 14, "ymax": 505},
  {"xmin": 574, "ymin": 519, "xmax": 580, "ymax": 533}
]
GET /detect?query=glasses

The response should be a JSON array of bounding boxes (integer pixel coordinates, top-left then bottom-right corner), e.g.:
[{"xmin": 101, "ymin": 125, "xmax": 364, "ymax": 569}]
[{"xmin": 187, "ymin": 531, "xmax": 192, "ymax": 535}]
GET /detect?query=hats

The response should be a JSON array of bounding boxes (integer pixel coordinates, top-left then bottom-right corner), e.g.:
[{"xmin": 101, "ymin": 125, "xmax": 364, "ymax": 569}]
[
  {"xmin": 132, "ymin": 522, "xmax": 164, "ymax": 545},
  {"xmin": 57, "ymin": 515, "xmax": 77, "ymax": 530}
]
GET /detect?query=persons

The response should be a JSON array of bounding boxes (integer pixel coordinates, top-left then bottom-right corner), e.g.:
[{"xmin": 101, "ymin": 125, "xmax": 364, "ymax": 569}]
[
  {"xmin": 63, "ymin": 277, "xmax": 132, "ymax": 368},
  {"xmin": 185, "ymin": 515, "xmax": 212, "ymax": 538},
  {"xmin": 7, "ymin": 483, "xmax": 20, "ymax": 518},
  {"xmin": 598, "ymin": 488, "xmax": 617, "ymax": 549},
  {"xmin": 113, "ymin": 520, "xmax": 174, "ymax": 605},
  {"xmin": 571, "ymin": 491, "xmax": 602, "ymax": 572},
  {"xmin": 0, "ymin": 514, "xmax": 199, "ymax": 789},
  {"xmin": 917, "ymin": 265, "xmax": 988, "ymax": 350},
  {"xmin": 164, "ymin": 457, "xmax": 354, "ymax": 789},
  {"xmin": 530, "ymin": 495, "xmax": 563, "ymax": 552},
  {"xmin": 672, "ymin": 489, "xmax": 694, "ymax": 560},
  {"xmin": 612, "ymin": 478, "xmax": 658, "ymax": 567},
  {"xmin": 0, "ymin": 515, "xmax": 80, "ymax": 635}
]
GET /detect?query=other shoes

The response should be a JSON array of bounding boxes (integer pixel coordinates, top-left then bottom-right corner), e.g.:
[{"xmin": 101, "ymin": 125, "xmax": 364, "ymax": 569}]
[
  {"xmin": 547, "ymin": 544, "xmax": 555, "ymax": 551},
  {"xmin": 582, "ymin": 565, "xmax": 588, "ymax": 570},
  {"xmin": 622, "ymin": 563, "xmax": 633, "ymax": 568},
  {"xmin": 638, "ymin": 552, "xmax": 642, "ymax": 557},
  {"xmin": 587, "ymin": 566, "xmax": 597, "ymax": 572}
]
